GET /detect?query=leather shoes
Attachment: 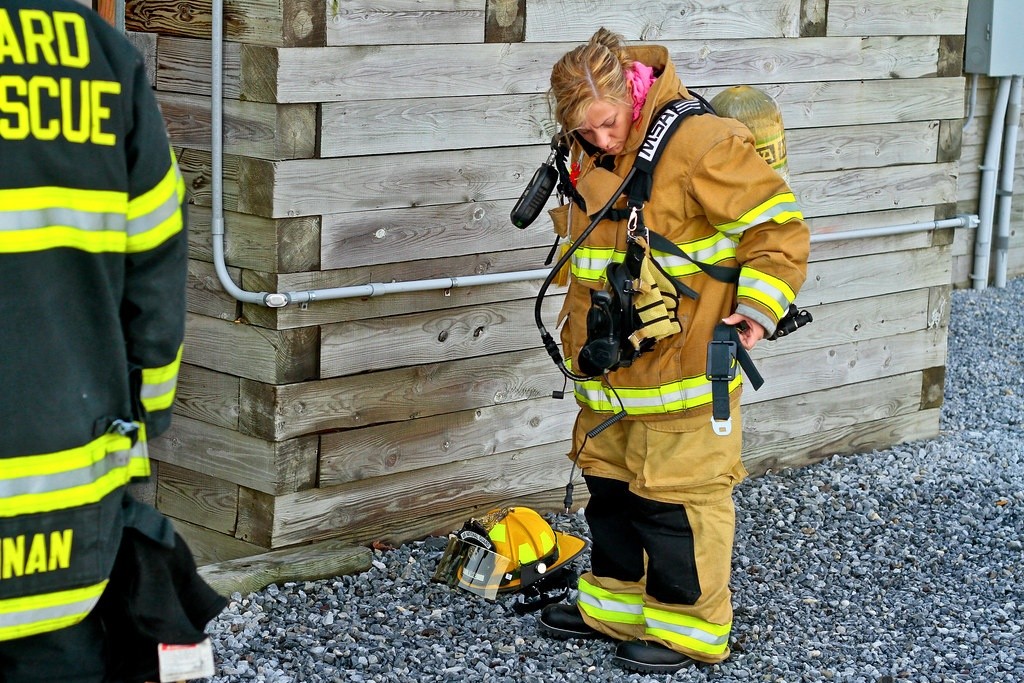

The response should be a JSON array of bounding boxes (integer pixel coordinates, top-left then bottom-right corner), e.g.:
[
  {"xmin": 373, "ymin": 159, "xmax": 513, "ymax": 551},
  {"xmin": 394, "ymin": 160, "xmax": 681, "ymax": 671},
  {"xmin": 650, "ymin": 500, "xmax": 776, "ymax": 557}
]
[
  {"xmin": 611, "ymin": 638, "xmax": 696, "ymax": 675},
  {"xmin": 538, "ymin": 603, "xmax": 602, "ymax": 639}
]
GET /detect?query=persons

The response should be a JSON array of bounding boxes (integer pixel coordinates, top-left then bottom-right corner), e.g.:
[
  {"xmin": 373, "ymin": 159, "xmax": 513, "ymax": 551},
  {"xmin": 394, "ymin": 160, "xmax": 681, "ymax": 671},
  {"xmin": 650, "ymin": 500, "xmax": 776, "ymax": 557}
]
[
  {"xmin": 0, "ymin": 0, "xmax": 188, "ymax": 683},
  {"xmin": 535, "ymin": 25, "xmax": 810, "ymax": 673}
]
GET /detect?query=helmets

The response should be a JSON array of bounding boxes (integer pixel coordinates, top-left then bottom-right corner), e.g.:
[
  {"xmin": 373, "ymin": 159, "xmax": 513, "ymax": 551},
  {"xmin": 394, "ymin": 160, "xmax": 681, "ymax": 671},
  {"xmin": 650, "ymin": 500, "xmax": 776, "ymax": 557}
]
[{"xmin": 436, "ymin": 506, "xmax": 591, "ymax": 601}]
